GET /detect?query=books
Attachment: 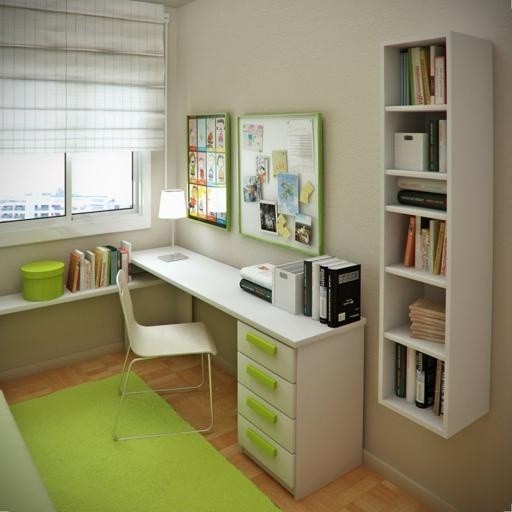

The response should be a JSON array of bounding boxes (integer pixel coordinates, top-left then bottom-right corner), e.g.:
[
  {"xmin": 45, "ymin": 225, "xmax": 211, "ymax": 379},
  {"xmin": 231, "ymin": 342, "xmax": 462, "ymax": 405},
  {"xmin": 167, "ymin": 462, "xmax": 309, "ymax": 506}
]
[
  {"xmin": 239, "ymin": 262, "xmax": 276, "ymax": 292},
  {"xmin": 237, "ymin": 278, "xmax": 273, "ymax": 304},
  {"xmin": 392, "ymin": 42, "xmax": 447, "ymax": 420},
  {"xmin": 65, "ymin": 239, "xmax": 132, "ymax": 293},
  {"xmin": 301, "ymin": 255, "xmax": 361, "ymax": 328}
]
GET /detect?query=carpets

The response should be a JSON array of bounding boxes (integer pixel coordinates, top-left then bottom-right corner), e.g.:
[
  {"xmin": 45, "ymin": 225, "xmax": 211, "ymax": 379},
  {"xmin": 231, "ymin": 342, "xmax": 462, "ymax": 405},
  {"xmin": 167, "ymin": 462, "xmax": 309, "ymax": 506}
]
[{"xmin": 9, "ymin": 372, "xmax": 281, "ymax": 512}]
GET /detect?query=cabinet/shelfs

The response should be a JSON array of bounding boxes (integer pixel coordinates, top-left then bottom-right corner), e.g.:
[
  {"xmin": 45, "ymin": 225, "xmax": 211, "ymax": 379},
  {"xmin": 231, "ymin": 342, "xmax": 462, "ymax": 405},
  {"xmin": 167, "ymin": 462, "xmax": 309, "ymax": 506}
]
[{"xmin": 377, "ymin": 31, "xmax": 495, "ymax": 440}]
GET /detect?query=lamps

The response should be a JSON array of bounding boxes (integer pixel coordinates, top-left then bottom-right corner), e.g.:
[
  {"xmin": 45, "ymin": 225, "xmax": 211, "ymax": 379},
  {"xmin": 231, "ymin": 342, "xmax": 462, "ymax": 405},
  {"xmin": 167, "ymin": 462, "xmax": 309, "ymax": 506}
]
[{"xmin": 158, "ymin": 189, "xmax": 188, "ymax": 263}]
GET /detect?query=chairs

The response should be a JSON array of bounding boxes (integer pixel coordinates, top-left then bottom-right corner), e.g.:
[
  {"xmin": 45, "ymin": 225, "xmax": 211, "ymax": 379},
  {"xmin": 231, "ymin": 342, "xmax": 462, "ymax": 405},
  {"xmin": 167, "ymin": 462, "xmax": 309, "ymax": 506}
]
[{"xmin": 112, "ymin": 269, "xmax": 217, "ymax": 441}]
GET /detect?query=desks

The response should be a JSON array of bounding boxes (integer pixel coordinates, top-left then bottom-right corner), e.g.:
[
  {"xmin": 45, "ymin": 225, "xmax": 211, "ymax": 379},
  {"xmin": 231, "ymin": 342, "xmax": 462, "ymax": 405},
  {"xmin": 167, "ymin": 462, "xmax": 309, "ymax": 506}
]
[
  {"xmin": 129, "ymin": 244, "xmax": 368, "ymax": 501},
  {"xmin": 0, "ymin": 272, "xmax": 194, "ymax": 324}
]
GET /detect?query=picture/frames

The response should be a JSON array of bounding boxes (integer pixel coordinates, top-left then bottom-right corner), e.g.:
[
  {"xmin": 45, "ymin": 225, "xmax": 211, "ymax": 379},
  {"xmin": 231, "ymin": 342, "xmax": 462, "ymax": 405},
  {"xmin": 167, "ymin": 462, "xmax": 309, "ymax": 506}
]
[
  {"xmin": 186, "ymin": 112, "xmax": 231, "ymax": 231},
  {"xmin": 236, "ymin": 112, "xmax": 324, "ymax": 256}
]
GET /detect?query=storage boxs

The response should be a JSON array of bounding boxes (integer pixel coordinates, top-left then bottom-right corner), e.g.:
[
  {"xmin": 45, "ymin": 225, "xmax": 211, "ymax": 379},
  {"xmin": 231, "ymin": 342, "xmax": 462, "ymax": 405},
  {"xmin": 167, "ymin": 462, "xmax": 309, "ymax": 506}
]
[
  {"xmin": 21, "ymin": 261, "xmax": 64, "ymax": 301},
  {"xmin": 394, "ymin": 132, "xmax": 430, "ymax": 171}
]
